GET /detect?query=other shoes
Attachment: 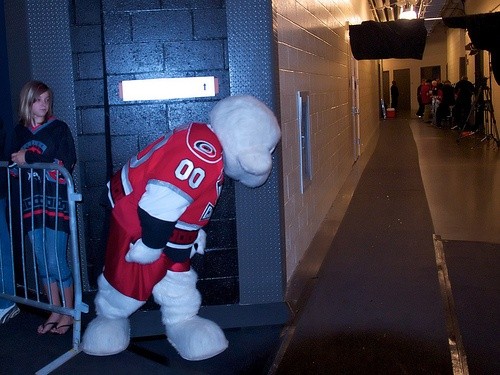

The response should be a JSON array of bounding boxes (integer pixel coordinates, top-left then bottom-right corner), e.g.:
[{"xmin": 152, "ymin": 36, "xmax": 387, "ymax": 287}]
[{"xmin": 0, "ymin": 303, "xmax": 20, "ymax": 325}]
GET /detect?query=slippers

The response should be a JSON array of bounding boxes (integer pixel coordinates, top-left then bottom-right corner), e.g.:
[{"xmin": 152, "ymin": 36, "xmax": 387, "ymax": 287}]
[
  {"xmin": 54, "ymin": 323, "xmax": 73, "ymax": 335},
  {"xmin": 35, "ymin": 321, "xmax": 57, "ymax": 336}
]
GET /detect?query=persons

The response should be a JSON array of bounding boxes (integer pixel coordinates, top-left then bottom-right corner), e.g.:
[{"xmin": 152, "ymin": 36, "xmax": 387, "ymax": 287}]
[
  {"xmin": 4, "ymin": 80, "xmax": 75, "ymax": 335},
  {"xmin": 390, "ymin": 75, "xmax": 484, "ymax": 132}
]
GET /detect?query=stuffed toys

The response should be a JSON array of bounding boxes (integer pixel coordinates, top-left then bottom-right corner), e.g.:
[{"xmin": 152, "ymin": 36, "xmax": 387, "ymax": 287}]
[{"xmin": 80, "ymin": 94, "xmax": 282, "ymax": 361}]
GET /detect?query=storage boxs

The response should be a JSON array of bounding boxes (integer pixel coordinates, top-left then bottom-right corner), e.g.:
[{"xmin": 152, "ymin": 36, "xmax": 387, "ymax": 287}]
[{"xmin": 386, "ymin": 108, "xmax": 395, "ymax": 118}]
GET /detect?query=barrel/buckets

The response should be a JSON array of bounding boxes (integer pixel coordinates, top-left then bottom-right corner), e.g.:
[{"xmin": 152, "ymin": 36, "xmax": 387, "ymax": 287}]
[{"xmin": 386, "ymin": 108, "xmax": 395, "ymax": 117}]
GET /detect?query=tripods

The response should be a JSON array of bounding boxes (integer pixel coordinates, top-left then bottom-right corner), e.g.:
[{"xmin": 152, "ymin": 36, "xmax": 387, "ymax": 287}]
[{"xmin": 455, "ymin": 78, "xmax": 500, "ymax": 149}]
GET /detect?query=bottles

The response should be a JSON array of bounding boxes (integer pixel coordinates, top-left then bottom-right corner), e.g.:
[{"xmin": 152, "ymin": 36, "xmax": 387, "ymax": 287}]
[{"xmin": 434, "ymin": 87, "xmax": 437, "ymax": 95}]
